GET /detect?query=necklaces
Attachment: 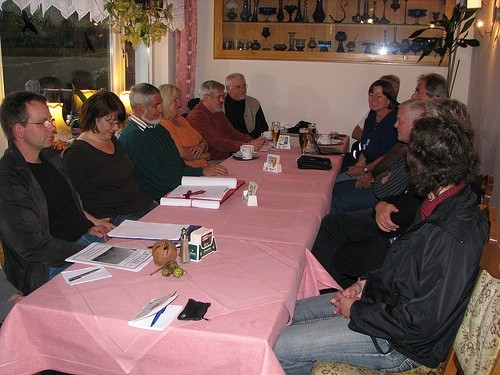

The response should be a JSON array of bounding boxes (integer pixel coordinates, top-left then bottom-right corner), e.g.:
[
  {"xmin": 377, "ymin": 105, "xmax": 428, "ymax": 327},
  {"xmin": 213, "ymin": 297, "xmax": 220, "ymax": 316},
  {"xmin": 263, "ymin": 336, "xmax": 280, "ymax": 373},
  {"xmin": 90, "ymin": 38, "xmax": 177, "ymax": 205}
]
[{"xmin": 428, "ymin": 186, "xmax": 444, "ymax": 200}]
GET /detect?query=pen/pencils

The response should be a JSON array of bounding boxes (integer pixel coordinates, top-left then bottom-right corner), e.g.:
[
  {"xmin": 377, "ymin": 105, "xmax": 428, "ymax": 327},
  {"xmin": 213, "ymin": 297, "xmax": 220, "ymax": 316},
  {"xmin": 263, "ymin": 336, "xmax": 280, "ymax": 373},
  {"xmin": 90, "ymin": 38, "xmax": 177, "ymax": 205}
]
[
  {"xmin": 150, "ymin": 306, "xmax": 168, "ymax": 327},
  {"xmin": 147, "ymin": 243, "xmax": 183, "ymax": 249},
  {"xmin": 68, "ymin": 267, "xmax": 101, "ymax": 282}
]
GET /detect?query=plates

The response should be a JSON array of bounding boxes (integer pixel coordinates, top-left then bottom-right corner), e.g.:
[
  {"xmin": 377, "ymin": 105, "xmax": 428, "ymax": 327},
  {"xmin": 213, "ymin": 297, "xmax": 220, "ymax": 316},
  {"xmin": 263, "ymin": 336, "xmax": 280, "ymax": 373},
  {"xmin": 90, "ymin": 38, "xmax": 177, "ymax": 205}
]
[
  {"xmin": 318, "ymin": 135, "xmax": 341, "ymax": 145},
  {"xmin": 232, "ymin": 155, "xmax": 259, "ymax": 161}
]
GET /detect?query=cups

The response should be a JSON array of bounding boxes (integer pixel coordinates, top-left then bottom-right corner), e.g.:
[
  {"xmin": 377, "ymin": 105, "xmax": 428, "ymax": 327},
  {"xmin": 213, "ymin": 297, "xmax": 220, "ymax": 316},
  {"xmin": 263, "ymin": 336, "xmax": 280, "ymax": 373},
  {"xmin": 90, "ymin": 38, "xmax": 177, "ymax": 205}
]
[
  {"xmin": 261, "ymin": 131, "xmax": 272, "ymax": 140},
  {"xmin": 240, "ymin": 145, "xmax": 255, "ymax": 159},
  {"xmin": 308, "ymin": 123, "xmax": 317, "ymax": 143},
  {"xmin": 271, "ymin": 122, "xmax": 280, "ymax": 146},
  {"xmin": 330, "ymin": 132, "xmax": 338, "ymax": 139}
]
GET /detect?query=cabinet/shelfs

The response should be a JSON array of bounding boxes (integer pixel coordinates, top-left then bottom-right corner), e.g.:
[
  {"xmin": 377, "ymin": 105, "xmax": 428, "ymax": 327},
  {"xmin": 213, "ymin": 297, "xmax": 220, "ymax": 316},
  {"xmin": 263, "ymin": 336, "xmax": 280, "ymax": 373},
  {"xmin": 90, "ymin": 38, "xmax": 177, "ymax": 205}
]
[{"xmin": 212, "ymin": 0, "xmax": 457, "ymax": 68}]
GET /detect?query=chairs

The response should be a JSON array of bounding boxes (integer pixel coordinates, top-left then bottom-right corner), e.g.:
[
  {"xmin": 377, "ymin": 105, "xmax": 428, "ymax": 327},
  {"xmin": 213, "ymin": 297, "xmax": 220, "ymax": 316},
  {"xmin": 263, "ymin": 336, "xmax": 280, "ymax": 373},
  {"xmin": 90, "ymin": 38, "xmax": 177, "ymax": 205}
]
[{"xmin": 311, "ymin": 154, "xmax": 500, "ymax": 375}]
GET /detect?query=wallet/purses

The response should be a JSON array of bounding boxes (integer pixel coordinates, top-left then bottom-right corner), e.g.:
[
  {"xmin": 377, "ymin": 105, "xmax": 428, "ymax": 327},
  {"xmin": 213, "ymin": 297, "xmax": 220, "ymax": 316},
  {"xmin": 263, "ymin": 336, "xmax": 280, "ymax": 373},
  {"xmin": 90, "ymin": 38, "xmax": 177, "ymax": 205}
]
[{"xmin": 297, "ymin": 155, "xmax": 333, "ymax": 171}]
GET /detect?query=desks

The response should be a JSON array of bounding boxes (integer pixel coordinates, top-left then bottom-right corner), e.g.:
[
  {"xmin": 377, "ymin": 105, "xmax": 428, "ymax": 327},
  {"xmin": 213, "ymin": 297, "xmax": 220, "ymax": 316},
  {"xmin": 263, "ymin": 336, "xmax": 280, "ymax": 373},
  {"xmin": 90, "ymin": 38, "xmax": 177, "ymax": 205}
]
[{"xmin": 0, "ymin": 128, "xmax": 350, "ymax": 375}]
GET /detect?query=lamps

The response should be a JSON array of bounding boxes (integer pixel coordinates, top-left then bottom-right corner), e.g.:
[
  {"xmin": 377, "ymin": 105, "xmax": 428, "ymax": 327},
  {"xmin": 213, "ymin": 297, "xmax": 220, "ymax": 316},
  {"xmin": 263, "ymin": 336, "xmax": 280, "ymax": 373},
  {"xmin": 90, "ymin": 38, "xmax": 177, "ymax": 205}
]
[{"xmin": 476, "ymin": 19, "xmax": 492, "ymax": 37}]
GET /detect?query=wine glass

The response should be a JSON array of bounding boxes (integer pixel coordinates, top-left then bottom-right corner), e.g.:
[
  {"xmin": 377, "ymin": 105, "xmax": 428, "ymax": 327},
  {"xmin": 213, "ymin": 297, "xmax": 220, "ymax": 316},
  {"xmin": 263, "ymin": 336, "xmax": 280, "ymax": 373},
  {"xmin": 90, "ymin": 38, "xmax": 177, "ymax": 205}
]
[{"xmin": 299, "ymin": 128, "xmax": 308, "ymax": 156}]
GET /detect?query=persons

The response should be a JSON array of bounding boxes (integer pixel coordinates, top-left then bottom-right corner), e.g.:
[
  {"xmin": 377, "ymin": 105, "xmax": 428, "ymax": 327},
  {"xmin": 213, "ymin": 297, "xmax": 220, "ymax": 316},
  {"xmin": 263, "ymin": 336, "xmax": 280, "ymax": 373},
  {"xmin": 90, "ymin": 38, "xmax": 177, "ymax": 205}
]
[
  {"xmin": 158, "ymin": 84, "xmax": 211, "ymax": 168},
  {"xmin": 62, "ymin": 90, "xmax": 160, "ymax": 232},
  {"xmin": 117, "ymin": 83, "xmax": 228, "ymax": 204},
  {"xmin": 273, "ymin": 116, "xmax": 492, "ymax": 375},
  {"xmin": 224, "ymin": 73, "xmax": 269, "ymax": 140},
  {"xmin": 186, "ymin": 80, "xmax": 269, "ymax": 161},
  {"xmin": 0, "ymin": 90, "xmax": 112, "ymax": 324},
  {"xmin": 311, "ymin": 73, "xmax": 470, "ymax": 294}
]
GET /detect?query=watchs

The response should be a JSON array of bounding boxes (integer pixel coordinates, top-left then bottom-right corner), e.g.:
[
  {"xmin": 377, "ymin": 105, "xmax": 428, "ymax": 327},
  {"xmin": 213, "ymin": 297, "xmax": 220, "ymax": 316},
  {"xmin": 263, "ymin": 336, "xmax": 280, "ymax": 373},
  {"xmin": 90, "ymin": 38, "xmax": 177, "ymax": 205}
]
[{"xmin": 363, "ymin": 166, "xmax": 369, "ymax": 173}]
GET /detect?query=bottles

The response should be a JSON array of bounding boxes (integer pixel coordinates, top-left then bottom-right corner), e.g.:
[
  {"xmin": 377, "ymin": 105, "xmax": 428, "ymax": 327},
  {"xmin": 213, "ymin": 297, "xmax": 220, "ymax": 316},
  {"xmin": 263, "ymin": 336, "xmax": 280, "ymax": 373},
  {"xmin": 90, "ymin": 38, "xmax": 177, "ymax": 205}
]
[
  {"xmin": 276, "ymin": 0, "xmax": 285, "ymax": 22},
  {"xmin": 180, "ymin": 227, "xmax": 190, "ymax": 264},
  {"xmin": 240, "ymin": 0, "xmax": 250, "ymax": 22},
  {"xmin": 312, "ymin": 0, "xmax": 325, "ymax": 23},
  {"xmin": 223, "ymin": 40, "xmax": 261, "ymax": 50}
]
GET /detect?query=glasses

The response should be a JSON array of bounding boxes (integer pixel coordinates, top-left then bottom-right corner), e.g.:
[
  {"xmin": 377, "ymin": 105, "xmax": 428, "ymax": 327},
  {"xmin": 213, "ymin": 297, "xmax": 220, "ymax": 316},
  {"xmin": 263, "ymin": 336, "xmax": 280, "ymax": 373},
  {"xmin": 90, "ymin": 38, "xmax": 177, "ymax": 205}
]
[
  {"xmin": 102, "ymin": 117, "xmax": 124, "ymax": 127},
  {"xmin": 205, "ymin": 93, "xmax": 226, "ymax": 101},
  {"xmin": 16, "ymin": 117, "xmax": 57, "ymax": 127},
  {"xmin": 230, "ymin": 84, "xmax": 248, "ymax": 89}
]
